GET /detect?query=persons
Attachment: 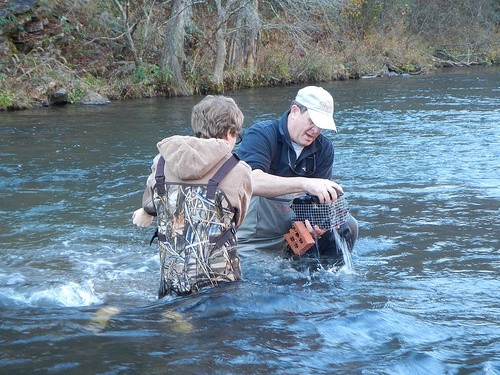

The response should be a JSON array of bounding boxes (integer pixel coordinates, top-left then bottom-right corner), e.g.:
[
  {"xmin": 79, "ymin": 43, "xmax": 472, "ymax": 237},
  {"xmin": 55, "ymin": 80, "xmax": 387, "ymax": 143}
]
[
  {"xmin": 236, "ymin": 85, "xmax": 358, "ymax": 267},
  {"xmin": 129, "ymin": 95, "xmax": 252, "ymax": 298}
]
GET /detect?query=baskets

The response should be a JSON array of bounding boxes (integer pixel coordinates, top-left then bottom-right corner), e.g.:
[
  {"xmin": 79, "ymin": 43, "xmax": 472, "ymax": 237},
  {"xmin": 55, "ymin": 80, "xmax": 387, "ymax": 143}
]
[{"xmin": 289, "ymin": 188, "xmax": 351, "ymax": 231}]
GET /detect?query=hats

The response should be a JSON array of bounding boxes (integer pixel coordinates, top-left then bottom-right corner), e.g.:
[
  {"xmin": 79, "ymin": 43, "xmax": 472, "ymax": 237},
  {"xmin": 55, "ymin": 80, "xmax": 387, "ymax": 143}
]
[{"xmin": 295, "ymin": 84, "xmax": 339, "ymax": 132}]
